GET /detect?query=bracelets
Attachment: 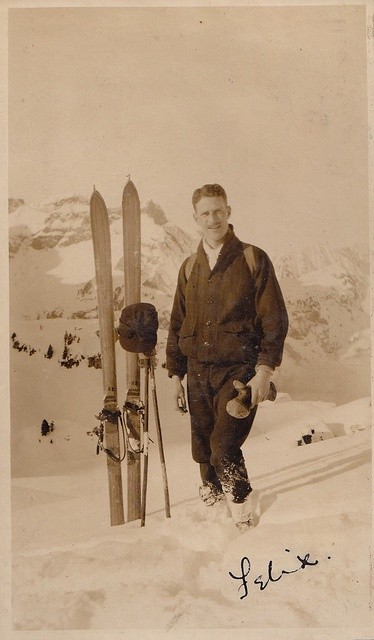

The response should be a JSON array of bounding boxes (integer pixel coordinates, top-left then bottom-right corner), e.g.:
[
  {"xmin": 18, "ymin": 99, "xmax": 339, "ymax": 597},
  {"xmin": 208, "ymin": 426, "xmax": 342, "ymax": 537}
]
[{"xmin": 256, "ymin": 364, "xmax": 274, "ymax": 375}]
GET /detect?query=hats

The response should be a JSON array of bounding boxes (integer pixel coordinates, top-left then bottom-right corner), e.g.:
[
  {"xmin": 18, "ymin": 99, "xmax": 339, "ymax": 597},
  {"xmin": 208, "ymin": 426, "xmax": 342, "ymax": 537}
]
[{"xmin": 118, "ymin": 303, "xmax": 158, "ymax": 353}]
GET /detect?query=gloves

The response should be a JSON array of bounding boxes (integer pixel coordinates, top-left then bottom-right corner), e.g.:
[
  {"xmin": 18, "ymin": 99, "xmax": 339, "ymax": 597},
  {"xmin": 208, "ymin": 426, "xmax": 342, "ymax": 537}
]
[{"xmin": 226, "ymin": 379, "xmax": 277, "ymax": 419}]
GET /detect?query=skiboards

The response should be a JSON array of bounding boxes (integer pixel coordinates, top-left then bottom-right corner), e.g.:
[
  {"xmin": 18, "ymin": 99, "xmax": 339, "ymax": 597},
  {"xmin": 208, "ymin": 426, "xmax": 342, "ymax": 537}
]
[{"xmin": 89, "ymin": 174, "xmax": 140, "ymax": 525}]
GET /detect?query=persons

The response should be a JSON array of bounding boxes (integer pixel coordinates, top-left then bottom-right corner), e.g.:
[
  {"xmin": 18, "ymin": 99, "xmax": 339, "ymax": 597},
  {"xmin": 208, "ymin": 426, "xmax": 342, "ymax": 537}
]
[{"xmin": 165, "ymin": 183, "xmax": 289, "ymax": 532}]
